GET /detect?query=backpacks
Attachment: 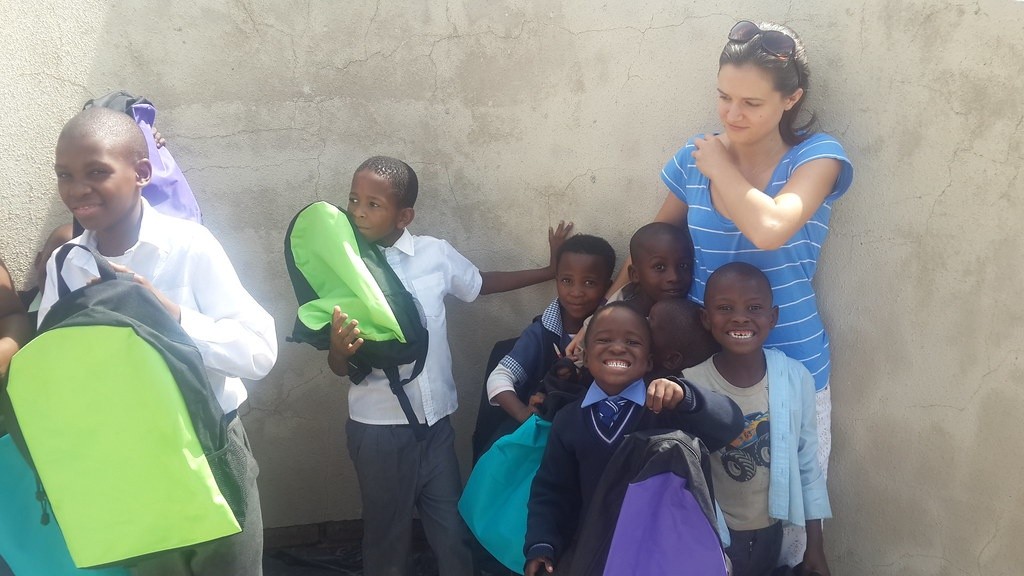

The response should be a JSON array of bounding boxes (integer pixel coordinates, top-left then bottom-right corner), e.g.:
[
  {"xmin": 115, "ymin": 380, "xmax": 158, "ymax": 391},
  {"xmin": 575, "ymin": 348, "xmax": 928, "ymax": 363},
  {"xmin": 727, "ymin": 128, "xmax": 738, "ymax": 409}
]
[
  {"xmin": 457, "ymin": 358, "xmax": 584, "ymax": 576},
  {"xmin": 284, "ymin": 200, "xmax": 429, "ymax": 385},
  {"xmin": 0, "ymin": 430, "xmax": 126, "ymax": 576},
  {"xmin": 0, "ymin": 241, "xmax": 247, "ymax": 568},
  {"xmin": 560, "ymin": 404, "xmax": 733, "ymax": 576},
  {"xmin": 73, "ymin": 89, "xmax": 203, "ymax": 240},
  {"xmin": 472, "ymin": 314, "xmax": 560, "ymax": 468}
]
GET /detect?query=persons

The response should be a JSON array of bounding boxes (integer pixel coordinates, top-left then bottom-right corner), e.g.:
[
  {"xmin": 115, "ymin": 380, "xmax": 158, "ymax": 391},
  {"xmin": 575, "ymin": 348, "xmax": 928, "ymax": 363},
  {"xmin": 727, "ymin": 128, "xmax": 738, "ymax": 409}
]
[
  {"xmin": 487, "ymin": 22, "xmax": 854, "ymax": 576},
  {"xmin": 0, "ymin": 107, "xmax": 278, "ymax": 575},
  {"xmin": 326, "ymin": 157, "xmax": 582, "ymax": 575}
]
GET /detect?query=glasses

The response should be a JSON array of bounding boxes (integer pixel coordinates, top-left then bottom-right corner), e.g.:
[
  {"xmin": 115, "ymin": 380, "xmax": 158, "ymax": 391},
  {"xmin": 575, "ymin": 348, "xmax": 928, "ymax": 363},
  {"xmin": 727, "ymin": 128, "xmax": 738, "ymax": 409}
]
[{"xmin": 728, "ymin": 21, "xmax": 801, "ymax": 89}]
